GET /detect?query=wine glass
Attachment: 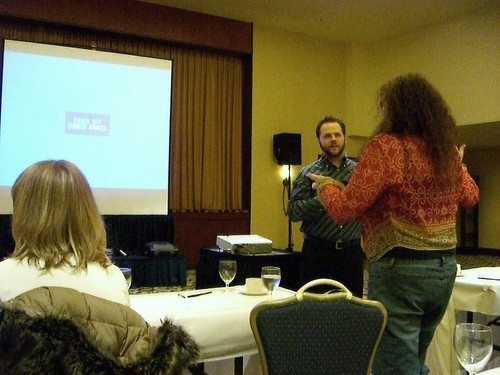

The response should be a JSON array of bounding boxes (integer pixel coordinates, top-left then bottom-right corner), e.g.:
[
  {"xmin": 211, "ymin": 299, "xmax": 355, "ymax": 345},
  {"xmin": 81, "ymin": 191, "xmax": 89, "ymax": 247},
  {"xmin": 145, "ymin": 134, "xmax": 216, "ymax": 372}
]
[
  {"xmin": 453, "ymin": 323, "xmax": 493, "ymax": 375},
  {"xmin": 219, "ymin": 260, "xmax": 237, "ymax": 294},
  {"xmin": 261, "ymin": 266, "xmax": 281, "ymax": 300}
]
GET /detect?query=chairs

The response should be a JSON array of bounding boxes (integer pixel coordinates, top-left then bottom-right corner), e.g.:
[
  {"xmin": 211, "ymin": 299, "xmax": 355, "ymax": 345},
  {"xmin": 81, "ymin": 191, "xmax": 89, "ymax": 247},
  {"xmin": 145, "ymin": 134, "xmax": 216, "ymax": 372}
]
[
  {"xmin": 422, "ymin": 286, "xmax": 462, "ymax": 375},
  {"xmin": 0, "ymin": 286, "xmax": 202, "ymax": 375},
  {"xmin": 249, "ymin": 277, "xmax": 389, "ymax": 375}
]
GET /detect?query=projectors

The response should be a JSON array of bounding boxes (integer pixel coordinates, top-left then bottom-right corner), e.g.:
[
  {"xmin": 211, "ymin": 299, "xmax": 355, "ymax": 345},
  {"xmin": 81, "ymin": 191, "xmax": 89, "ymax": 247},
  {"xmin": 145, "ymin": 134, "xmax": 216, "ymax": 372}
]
[{"xmin": 216, "ymin": 235, "xmax": 273, "ymax": 255}]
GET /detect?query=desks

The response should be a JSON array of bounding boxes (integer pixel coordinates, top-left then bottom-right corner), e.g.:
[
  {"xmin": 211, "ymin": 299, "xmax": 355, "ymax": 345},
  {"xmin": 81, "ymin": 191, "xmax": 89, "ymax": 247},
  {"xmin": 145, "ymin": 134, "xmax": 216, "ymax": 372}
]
[
  {"xmin": 451, "ymin": 264, "xmax": 500, "ymax": 375},
  {"xmin": 127, "ymin": 281, "xmax": 297, "ymax": 375},
  {"xmin": 109, "ymin": 252, "xmax": 188, "ymax": 289},
  {"xmin": 195, "ymin": 247, "xmax": 301, "ymax": 293}
]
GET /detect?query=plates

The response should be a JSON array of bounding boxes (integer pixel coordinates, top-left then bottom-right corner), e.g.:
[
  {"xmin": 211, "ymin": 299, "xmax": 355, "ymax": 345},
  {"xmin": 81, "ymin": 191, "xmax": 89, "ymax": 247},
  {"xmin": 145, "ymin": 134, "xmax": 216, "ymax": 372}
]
[{"xmin": 240, "ymin": 290, "xmax": 267, "ymax": 295}]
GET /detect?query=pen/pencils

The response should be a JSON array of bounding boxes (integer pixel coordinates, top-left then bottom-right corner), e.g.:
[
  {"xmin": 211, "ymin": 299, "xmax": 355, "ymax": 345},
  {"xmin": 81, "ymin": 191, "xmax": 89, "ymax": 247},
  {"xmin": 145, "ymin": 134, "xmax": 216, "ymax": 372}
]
[
  {"xmin": 188, "ymin": 290, "xmax": 213, "ymax": 298},
  {"xmin": 177, "ymin": 293, "xmax": 186, "ymax": 298},
  {"xmin": 478, "ymin": 276, "xmax": 500, "ymax": 281}
]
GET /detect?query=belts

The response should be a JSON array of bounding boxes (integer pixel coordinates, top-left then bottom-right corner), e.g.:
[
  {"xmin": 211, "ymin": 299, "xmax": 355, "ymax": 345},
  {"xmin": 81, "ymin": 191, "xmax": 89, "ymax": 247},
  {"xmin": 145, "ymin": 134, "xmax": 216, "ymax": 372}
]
[{"xmin": 303, "ymin": 233, "xmax": 361, "ymax": 250}]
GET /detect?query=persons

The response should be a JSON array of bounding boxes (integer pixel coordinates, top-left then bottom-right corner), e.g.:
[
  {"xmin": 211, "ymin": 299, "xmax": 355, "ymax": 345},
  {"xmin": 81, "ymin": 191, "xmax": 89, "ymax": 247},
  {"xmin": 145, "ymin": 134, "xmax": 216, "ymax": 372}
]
[
  {"xmin": 0, "ymin": 160, "xmax": 132, "ymax": 305},
  {"xmin": 288, "ymin": 116, "xmax": 365, "ymax": 297},
  {"xmin": 307, "ymin": 71, "xmax": 480, "ymax": 375}
]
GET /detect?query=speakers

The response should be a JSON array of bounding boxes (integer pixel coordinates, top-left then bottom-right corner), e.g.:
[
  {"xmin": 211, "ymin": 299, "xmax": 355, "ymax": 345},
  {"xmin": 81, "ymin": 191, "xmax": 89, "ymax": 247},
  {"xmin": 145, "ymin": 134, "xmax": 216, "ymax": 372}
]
[{"xmin": 274, "ymin": 132, "xmax": 301, "ymax": 165}]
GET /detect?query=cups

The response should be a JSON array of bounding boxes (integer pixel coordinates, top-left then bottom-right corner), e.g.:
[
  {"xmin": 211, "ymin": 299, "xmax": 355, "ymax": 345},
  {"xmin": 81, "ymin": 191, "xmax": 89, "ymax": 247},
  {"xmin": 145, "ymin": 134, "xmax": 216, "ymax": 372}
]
[
  {"xmin": 120, "ymin": 268, "xmax": 131, "ymax": 289},
  {"xmin": 246, "ymin": 278, "xmax": 263, "ymax": 293},
  {"xmin": 456, "ymin": 264, "xmax": 461, "ymax": 275}
]
